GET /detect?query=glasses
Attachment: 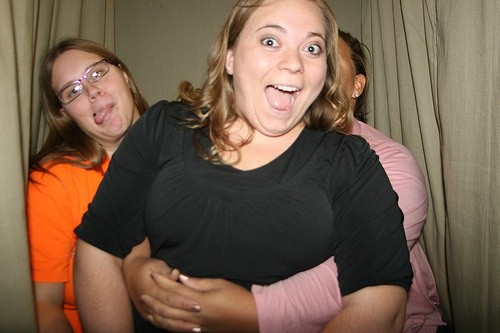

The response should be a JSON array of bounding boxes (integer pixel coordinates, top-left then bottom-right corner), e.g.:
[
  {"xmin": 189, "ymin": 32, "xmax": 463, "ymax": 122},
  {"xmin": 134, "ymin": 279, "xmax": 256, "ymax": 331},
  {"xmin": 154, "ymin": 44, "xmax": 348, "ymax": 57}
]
[{"xmin": 54, "ymin": 59, "xmax": 110, "ymax": 108}]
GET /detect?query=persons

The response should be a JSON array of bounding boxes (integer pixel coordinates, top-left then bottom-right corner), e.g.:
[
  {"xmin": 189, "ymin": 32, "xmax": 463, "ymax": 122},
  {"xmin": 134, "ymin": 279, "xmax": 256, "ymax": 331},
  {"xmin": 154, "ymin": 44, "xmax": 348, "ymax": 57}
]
[
  {"xmin": 72, "ymin": 0, "xmax": 416, "ymax": 333},
  {"xmin": 121, "ymin": 26, "xmax": 448, "ymax": 333},
  {"xmin": 27, "ymin": 36, "xmax": 150, "ymax": 332}
]
